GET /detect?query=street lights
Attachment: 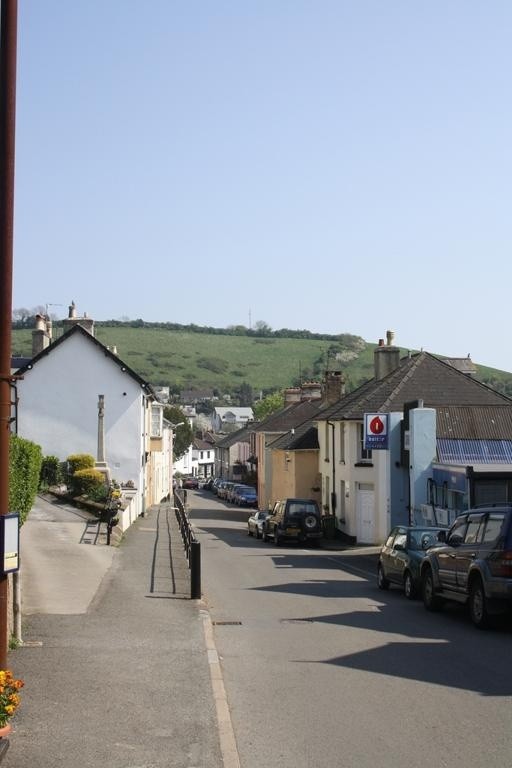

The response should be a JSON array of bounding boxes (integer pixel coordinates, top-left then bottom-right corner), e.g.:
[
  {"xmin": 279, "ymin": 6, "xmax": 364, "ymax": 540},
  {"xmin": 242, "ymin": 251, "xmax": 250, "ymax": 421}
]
[{"xmin": 169, "ymin": 421, "xmax": 186, "ymax": 500}]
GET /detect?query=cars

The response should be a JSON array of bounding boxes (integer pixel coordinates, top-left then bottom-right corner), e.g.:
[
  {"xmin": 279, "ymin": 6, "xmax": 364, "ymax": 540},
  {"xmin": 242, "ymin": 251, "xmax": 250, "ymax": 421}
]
[
  {"xmin": 183, "ymin": 477, "xmax": 198, "ymax": 489},
  {"xmin": 247, "ymin": 510, "xmax": 272, "ymax": 539},
  {"xmin": 204, "ymin": 477, "xmax": 257, "ymax": 508}
]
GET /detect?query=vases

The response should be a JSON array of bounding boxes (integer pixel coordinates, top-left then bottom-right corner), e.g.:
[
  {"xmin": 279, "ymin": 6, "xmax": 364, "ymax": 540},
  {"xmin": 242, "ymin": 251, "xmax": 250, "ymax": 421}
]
[{"xmin": 0, "ymin": 722, "xmax": 11, "ymax": 742}]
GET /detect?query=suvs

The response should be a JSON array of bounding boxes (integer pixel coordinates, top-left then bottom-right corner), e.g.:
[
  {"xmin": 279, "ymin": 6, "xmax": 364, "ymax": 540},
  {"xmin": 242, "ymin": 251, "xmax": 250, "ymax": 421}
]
[
  {"xmin": 378, "ymin": 525, "xmax": 451, "ymax": 602},
  {"xmin": 421, "ymin": 503, "xmax": 511, "ymax": 629},
  {"xmin": 262, "ymin": 498, "xmax": 324, "ymax": 548}
]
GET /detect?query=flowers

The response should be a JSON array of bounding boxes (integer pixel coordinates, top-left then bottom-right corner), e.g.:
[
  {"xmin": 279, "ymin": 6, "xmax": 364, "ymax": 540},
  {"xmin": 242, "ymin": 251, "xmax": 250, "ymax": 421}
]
[{"xmin": 0, "ymin": 669, "xmax": 25, "ymax": 726}]
